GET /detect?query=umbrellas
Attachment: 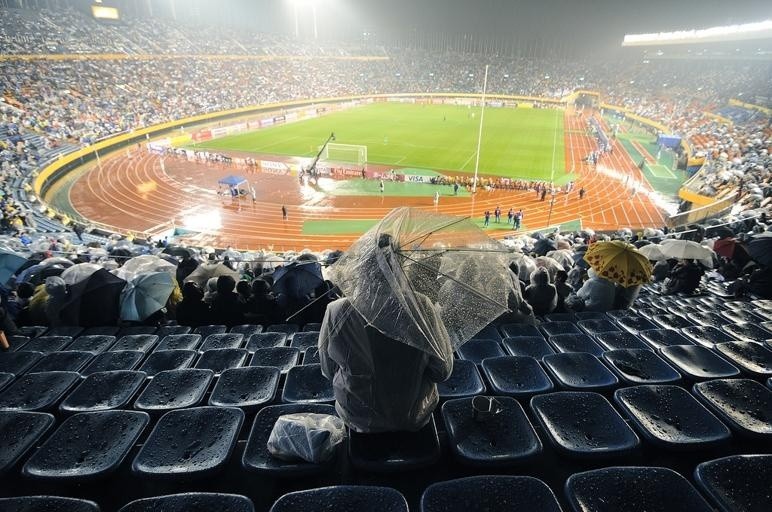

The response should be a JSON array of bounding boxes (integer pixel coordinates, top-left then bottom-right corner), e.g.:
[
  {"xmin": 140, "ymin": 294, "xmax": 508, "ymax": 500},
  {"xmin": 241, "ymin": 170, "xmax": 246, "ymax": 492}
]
[{"xmin": 322, "ymin": 204, "xmax": 516, "ymax": 359}]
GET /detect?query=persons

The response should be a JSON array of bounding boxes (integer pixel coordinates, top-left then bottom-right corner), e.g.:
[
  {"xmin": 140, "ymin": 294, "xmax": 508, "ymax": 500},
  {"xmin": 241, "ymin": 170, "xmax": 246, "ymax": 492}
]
[
  {"xmin": 162, "ymin": 146, "xmax": 259, "ymax": 173},
  {"xmin": 574, "ymin": 46, "xmax": 772, "ymax": 202},
  {"xmin": 0, "ymin": 201, "xmax": 771, "ymax": 326},
  {"xmin": 361, "ymin": 168, "xmax": 574, "ymax": 201},
  {"xmin": 251, "ymin": 187, "xmax": 257, "ymax": 204},
  {"xmin": 1, "ymin": 1, "xmax": 162, "ymax": 206},
  {"xmin": 307, "ymin": 233, "xmax": 457, "ymax": 436},
  {"xmin": 162, "ymin": 16, "xmax": 574, "ymax": 122}
]
